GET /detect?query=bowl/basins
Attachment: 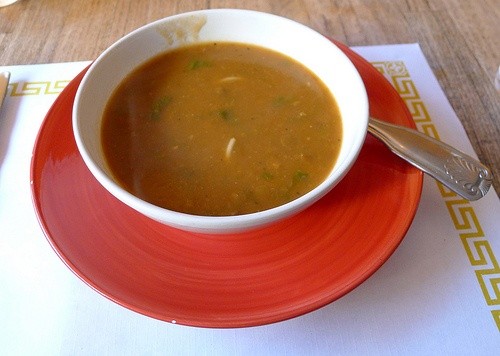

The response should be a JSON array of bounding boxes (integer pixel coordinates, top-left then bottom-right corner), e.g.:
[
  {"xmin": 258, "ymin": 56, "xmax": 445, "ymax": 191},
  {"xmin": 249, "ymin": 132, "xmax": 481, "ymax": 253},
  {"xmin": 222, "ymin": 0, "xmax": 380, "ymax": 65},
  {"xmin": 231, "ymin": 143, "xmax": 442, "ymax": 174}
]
[{"xmin": 71, "ymin": 9, "xmax": 370, "ymax": 235}]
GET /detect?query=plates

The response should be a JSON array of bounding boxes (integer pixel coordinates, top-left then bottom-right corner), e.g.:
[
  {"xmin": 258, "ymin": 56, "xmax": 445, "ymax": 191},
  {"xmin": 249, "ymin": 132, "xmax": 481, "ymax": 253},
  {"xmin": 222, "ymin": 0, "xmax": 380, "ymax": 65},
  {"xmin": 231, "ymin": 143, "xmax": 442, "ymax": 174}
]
[{"xmin": 30, "ymin": 35, "xmax": 424, "ymax": 329}]
